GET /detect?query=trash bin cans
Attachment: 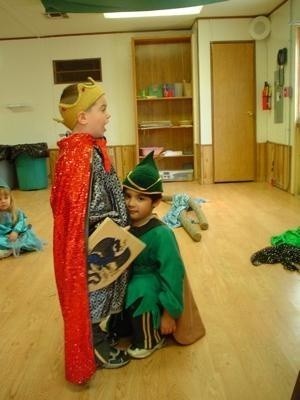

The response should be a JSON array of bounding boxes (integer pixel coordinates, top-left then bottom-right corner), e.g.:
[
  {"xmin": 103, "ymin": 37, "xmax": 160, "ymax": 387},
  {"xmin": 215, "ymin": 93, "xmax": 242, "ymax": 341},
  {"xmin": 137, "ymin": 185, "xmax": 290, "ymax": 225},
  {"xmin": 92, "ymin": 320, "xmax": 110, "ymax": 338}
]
[
  {"xmin": 9, "ymin": 142, "xmax": 49, "ymax": 191},
  {"xmin": 0, "ymin": 145, "xmax": 17, "ymax": 190}
]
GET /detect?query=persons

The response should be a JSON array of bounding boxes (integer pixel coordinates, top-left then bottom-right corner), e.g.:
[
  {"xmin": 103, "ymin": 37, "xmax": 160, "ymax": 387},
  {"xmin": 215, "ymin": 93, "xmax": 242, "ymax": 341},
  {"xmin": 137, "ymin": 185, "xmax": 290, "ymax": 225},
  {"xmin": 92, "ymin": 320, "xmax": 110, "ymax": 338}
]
[
  {"xmin": 0, "ymin": 178, "xmax": 44, "ymax": 260},
  {"xmin": 49, "ymin": 77, "xmax": 134, "ymax": 386},
  {"xmin": 120, "ymin": 147, "xmax": 207, "ymax": 360}
]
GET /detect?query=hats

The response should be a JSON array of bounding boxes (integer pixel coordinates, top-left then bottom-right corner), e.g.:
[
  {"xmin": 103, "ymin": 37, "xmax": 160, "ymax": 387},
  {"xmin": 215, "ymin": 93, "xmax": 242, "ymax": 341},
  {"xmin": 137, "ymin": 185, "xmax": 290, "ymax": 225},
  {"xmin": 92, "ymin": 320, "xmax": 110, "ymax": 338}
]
[{"xmin": 122, "ymin": 150, "xmax": 163, "ymax": 195}]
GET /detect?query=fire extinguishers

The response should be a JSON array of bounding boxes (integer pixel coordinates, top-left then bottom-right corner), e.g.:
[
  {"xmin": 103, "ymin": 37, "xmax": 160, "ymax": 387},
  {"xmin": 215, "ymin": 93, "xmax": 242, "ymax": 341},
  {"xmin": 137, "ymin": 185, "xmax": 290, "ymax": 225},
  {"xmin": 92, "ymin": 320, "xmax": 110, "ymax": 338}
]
[{"xmin": 262, "ymin": 82, "xmax": 269, "ymax": 110}]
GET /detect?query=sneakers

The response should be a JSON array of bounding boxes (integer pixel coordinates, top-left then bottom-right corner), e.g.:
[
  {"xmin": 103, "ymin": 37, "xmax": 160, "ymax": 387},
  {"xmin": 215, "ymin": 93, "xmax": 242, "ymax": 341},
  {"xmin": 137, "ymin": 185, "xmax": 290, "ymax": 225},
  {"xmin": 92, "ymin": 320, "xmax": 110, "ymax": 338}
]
[{"xmin": 93, "ymin": 314, "xmax": 167, "ymax": 369}]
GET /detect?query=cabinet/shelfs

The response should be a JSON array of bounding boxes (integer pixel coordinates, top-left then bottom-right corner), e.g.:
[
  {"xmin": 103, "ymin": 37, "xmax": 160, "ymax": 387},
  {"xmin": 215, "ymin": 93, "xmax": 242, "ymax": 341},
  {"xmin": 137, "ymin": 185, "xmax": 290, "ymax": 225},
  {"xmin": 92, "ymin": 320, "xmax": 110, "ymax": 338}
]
[{"xmin": 131, "ymin": 34, "xmax": 201, "ymax": 184}]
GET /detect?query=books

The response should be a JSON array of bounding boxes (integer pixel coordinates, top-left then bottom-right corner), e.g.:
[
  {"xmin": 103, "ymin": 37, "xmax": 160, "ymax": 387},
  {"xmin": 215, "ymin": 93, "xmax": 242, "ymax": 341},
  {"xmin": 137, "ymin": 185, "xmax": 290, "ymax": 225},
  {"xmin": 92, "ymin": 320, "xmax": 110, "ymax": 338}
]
[{"xmin": 138, "ymin": 118, "xmax": 173, "ymax": 130}]
